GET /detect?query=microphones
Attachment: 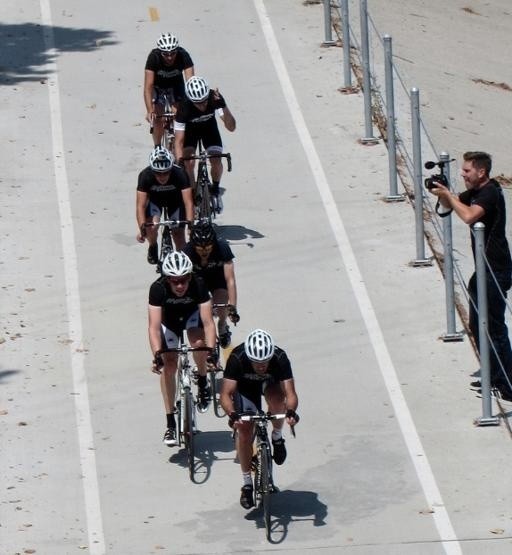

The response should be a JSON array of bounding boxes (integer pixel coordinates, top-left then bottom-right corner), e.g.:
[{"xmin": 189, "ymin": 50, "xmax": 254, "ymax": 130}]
[{"xmin": 424, "ymin": 159, "xmax": 456, "ymax": 169}]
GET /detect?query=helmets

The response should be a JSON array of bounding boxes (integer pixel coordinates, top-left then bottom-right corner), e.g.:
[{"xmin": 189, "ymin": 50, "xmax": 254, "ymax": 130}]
[
  {"xmin": 156, "ymin": 33, "xmax": 179, "ymax": 52},
  {"xmin": 191, "ymin": 222, "xmax": 216, "ymax": 245},
  {"xmin": 194, "ymin": 100, "xmax": 207, "ymax": 104},
  {"xmin": 196, "ymin": 247, "xmax": 212, "ymax": 250},
  {"xmin": 154, "ymin": 171, "xmax": 170, "ymax": 175},
  {"xmin": 245, "ymin": 329, "xmax": 274, "ymax": 363},
  {"xmin": 162, "ymin": 251, "xmax": 193, "ymax": 277},
  {"xmin": 185, "ymin": 76, "xmax": 210, "ymax": 103},
  {"xmin": 150, "ymin": 145, "xmax": 175, "ymax": 174},
  {"xmin": 168, "ymin": 279, "xmax": 187, "ymax": 284}
]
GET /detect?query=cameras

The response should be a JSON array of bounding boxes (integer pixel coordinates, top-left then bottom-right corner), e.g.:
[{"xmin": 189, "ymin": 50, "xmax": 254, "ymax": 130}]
[{"xmin": 425, "ymin": 174, "xmax": 450, "ymax": 190}]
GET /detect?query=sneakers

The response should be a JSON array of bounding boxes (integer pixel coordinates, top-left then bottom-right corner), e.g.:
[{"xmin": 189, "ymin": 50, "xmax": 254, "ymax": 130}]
[
  {"xmin": 272, "ymin": 436, "xmax": 286, "ymax": 465},
  {"xmin": 240, "ymin": 485, "xmax": 253, "ymax": 509},
  {"xmin": 197, "ymin": 385, "xmax": 208, "ymax": 413},
  {"xmin": 477, "ymin": 387, "xmax": 503, "ymax": 398},
  {"xmin": 470, "ymin": 380, "xmax": 499, "ymax": 390},
  {"xmin": 218, "ymin": 322, "xmax": 231, "ymax": 348},
  {"xmin": 214, "ymin": 194, "xmax": 222, "ymax": 213},
  {"xmin": 148, "ymin": 243, "xmax": 158, "ymax": 264},
  {"xmin": 163, "ymin": 428, "xmax": 176, "ymax": 444}
]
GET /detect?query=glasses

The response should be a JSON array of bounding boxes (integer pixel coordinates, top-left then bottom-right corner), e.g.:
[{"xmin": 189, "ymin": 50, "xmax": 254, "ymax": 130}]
[{"xmin": 163, "ymin": 52, "xmax": 176, "ymax": 57}]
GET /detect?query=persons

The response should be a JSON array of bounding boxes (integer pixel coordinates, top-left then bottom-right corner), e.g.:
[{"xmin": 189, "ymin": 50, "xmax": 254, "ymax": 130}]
[
  {"xmin": 147, "ymin": 250, "xmax": 218, "ymax": 448},
  {"xmin": 219, "ymin": 329, "xmax": 299, "ymax": 509},
  {"xmin": 428, "ymin": 150, "xmax": 512, "ymax": 403},
  {"xmin": 181, "ymin": 218, "xmax": 241, "ymax": 350},
  {"xmin": 133, "ymin": 146, "xmax": 196, "ymax": 265},
  {"xmin": 171, "ymin": 75, "xmax": 236, "ymax": 208},
  {"xmin": 144, "ymin": 31, "xmax": 195, "ymax": 152}
]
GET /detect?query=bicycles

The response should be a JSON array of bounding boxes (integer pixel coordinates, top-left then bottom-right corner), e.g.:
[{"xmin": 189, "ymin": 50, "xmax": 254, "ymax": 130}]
[
  {"xmin": 139, "ymin": 112, "xmax": 232, "ymax": 278},
  {"xmin": 227, "ymin": 412, "xmax": 296, "ymax": 540},
  {"xmin": 194, "ymin": 302, "xmax": 238, "ymax": 417},
  {"xmin": 150, "ymin": 328, "xmax": 217, "ymax": 463}
]
